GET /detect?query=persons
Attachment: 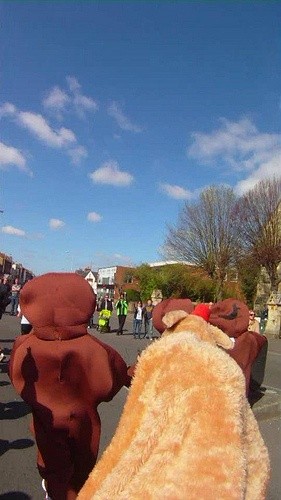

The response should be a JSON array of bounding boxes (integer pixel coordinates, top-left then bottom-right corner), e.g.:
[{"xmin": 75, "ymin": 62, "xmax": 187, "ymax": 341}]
[
  {"xmin": 0, "ymin": 273, "xmax": 33, "ymax": 362},
  {"xmin": 133, "ymin": 301, "xmax": 145, "ymax": 339},
  {"xmin": 247, "ymin": 309, "xmax": 261, "ymax": 335},
  {"xmin": 115, "ymin": 294, "xmax": 128, "ymax": 336},
  {"xmin": 87, "ymin": 294, "xmax": 114, "ymax": 328},
  {"xmin": 143, "ymin": 299, "xmax": 155, "ymax": 339}
]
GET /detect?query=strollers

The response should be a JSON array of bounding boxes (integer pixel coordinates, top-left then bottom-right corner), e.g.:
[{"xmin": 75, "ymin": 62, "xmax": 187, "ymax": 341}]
[{"xmin": 96, "ymin": 308, "xmax": 113, "ymax": 334}]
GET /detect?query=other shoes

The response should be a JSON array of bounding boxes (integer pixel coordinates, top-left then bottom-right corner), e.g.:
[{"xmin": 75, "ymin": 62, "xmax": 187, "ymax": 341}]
[
  {"xmin": 117, "ymin": 332, "xmax": 120, "ymax": 335},
  {"xmin": 88, "ymin": 326, "xmax": 90, "ymax": 328},
  {"xmin": 149, "ymin": 337, "xmax": 153, "ymax": 341},
  {"xmin": 10, "ymin": 312, "xmax": 13, "ymax": 315},
  {"xmin": 0, "ymin": 354, "xmax": 6, "ymax": 362},
  {"xmin": 121, "ymin": 331, "xmax": 122, "ymax": 334}
]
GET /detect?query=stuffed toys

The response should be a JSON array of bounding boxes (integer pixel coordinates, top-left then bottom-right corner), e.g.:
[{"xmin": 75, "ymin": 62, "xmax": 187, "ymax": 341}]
[
  {"xmin": 7, "ymin": 274, "xmax": 128, "ymax": 500},
  {"xmin": 126, "ymin": 297, "xmax": 194, "ymax": 390},
  {"xmin": 74, "ymin": 305, "xmax": 272, "ymax": 500},
  {"xmin": 208, "ymin": 297, "xmax": 268, "ymax": 405}
]
